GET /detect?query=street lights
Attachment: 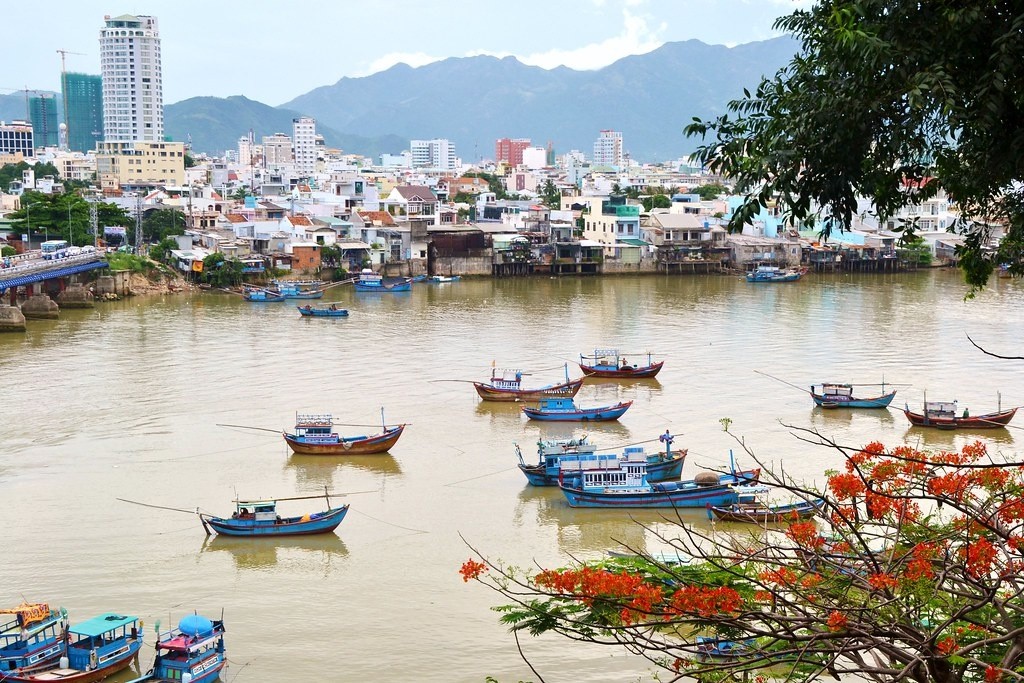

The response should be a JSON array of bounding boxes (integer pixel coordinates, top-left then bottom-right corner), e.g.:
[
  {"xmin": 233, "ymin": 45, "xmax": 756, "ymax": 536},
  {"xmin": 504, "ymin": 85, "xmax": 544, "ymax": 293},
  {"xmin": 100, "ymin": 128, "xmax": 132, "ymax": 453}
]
[
  {"xmin": 38, "ymin": 227, "xmax": 47, "ymax": 242},
  {"xmin": 69, "ymin": 200, "xmax": 81, "ymax": 247}
]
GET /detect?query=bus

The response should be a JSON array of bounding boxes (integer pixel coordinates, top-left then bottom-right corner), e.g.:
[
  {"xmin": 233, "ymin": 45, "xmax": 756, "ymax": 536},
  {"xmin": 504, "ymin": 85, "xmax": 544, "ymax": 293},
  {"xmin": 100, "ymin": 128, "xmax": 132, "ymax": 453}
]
[{"xmin": 40, "ymin": 240, "xmax": 68, "ymax": 261}]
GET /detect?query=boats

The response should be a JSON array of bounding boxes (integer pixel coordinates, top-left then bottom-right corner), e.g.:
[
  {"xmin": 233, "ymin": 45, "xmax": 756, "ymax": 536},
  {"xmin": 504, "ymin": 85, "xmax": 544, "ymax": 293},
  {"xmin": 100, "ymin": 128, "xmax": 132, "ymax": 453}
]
[
  {"xmin": 554, "ymin": 449, "xmax": 762, "ymax": 508},
  {"xmin": 602, "ymin": 546, "xmax": 702, "ymax": 593},
  {"xmin": 0, "ymin": 612, "xmax": 144, "ymax": 683},
  {"xmin": 297, "ymin": 301, "xmax": 350, "ymax": 317},
  {"xmin": 431, "ymin": 274, "xmax": 463, "ymax": 282},
  {"xmin": 122, "ymin": 614, "xmax": 227, "ymax": 683},
  {"xmin": 511, "ymin": 430, "xmax": 688, "ymax": 486},
  {"xmin": 274, "ymin": 281, "xmax": 325, "ymax": 299},
  {"xmin": 280, "ymin": 407, "xmax": 408, "ymax": 453},
  {"xmin": 704, "ymin": 483, "xmax": 830, "ymax": 522},
  {"xmin": 577, "ymin": 349, "xmax": 665, "ymax": 377},
  {"xmin": 350, "ymin": 270, "xmax": 412, "ymax": 292},
  {"xmin": 0, "ymin": 602, "xmax": 71, "ymax": 683},
  {"xmin": 902, "ymin": 390, "xmax": 1020, "ymax": 430},
  {"xmin": 791, "ymin": 546, "xmax": 889, "ymax": 584},
  {"xmin": 519, "ymin": 396, "xmax": 635, "ymax": 422},
  {"xmin": 473, "ymin": 366, "xmax": 596, "ymax": 403},
  {"xmin": 405, "ymin": 273, "xmax": 428, "ymax": 282},
  {"xmin": 746, "ymin": 263, "xmax": 810, "ymax": 282},
  {"xmin": 236, "ymin": 286, "xmax": 286, "ymax": 303},
  {"xmin": 195, "ymin": 489, "xmax": 351, "ymax": 535},
  {"xmin": 809, "ymin": 373, "xmax": 897, "ymax": 408},
  {"xmin": 692, "ymin": 635, "xmax": 818, "ymax": 662}
]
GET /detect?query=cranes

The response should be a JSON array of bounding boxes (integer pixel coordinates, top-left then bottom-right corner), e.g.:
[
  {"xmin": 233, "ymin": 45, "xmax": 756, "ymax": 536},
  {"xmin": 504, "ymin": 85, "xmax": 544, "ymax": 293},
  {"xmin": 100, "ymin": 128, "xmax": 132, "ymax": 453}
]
[
  {"xmin": 56, "ymin": 49, "xmax": 92, "ymax": 146},
  {"xmin": 0, "ymin": 84, "xmax": 50, "ymax": 123}
]
